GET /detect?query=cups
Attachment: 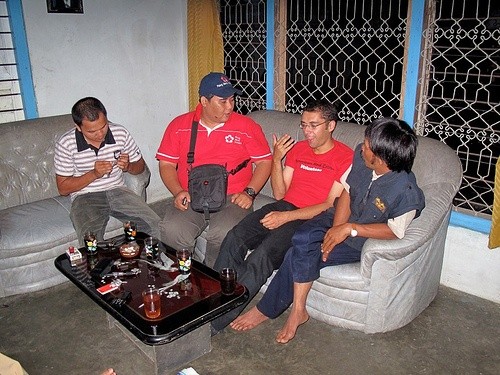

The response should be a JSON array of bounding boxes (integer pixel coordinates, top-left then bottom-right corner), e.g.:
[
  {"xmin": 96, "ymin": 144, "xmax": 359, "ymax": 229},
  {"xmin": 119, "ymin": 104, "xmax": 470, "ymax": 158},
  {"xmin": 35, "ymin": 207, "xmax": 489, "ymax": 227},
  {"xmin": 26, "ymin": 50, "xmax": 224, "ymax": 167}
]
[
  {"xmin": 83, "ymin": 230, "xmax": 98, "ymax": 255},
  {"xmin": 219, "ymin": 267, "xmax": 237, "ymax": 295},
  {"xmin": 144, "ymin": 236, "xmax": 160, "ymax": 263},
  {"xmin": 142, "ymin": 287, "xmax": 161, "ymax": 318},
  {"xmin": 123, "ymin": 221, "xmax": 138, "ymax": 244},
  {"xmin": 175, "ymin": 248, "xmax": 192, "ymax": 275}
]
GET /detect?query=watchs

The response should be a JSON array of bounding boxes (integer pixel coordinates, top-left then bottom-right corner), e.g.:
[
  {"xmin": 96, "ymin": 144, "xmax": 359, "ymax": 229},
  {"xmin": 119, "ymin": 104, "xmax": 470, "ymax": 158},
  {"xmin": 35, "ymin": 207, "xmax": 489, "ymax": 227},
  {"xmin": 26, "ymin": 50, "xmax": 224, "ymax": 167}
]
[
  {"xmin": 243, "ymin": 187, "xmax": 257, "ymax": 199},
  {"xmin": 350, "ymin": 223, "xmax": 357, "ymax": 238}
]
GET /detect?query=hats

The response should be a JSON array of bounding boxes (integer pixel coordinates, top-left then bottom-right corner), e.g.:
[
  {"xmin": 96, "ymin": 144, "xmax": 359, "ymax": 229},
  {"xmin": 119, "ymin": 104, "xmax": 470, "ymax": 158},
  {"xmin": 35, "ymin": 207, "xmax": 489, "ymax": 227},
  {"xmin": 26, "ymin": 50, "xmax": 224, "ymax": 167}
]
[{"xmin": 199, "ymin": 73, "xmax": 242, "ymax": 99}]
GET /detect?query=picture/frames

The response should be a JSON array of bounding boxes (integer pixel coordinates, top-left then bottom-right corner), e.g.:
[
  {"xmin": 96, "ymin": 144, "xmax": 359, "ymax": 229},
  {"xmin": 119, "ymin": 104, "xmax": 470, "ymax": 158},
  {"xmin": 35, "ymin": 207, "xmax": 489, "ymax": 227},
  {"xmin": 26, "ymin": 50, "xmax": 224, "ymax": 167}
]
[{"xmin": 46, "ymin": 0, "xmax": 84, "ymax": 14}]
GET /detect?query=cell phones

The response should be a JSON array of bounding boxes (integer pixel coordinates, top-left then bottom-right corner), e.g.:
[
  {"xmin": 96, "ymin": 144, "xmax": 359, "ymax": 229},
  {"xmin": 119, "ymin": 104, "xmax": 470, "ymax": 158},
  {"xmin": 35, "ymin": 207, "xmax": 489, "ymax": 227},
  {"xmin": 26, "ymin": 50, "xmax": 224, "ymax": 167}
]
[
  {"xmin": 182, "ymin": 197, "xmax": 186, "ymax": 205},
  {"xmin": 110, "ymin": 290, "xmax": 131, "ymax": 306}
]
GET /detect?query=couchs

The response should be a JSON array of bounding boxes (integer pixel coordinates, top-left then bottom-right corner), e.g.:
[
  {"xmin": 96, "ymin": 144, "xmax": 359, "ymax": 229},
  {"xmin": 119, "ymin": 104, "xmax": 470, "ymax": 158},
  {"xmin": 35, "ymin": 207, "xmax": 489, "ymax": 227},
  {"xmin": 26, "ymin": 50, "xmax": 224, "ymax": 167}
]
[
  {"xmin": 193, "ymin": 111, "xmax": 463, "ymax": 335},
  {"xmin": 0, "ymin": 114, "xmax": 150, "ymax": 299}
]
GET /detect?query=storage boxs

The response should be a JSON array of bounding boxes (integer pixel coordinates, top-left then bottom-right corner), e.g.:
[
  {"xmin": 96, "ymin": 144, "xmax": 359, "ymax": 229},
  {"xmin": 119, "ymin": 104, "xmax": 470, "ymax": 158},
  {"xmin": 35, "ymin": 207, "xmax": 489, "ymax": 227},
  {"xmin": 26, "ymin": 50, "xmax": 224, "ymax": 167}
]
[{"xmin": 65, "ymin": 248, "xmax": 83, "ymax": 261}]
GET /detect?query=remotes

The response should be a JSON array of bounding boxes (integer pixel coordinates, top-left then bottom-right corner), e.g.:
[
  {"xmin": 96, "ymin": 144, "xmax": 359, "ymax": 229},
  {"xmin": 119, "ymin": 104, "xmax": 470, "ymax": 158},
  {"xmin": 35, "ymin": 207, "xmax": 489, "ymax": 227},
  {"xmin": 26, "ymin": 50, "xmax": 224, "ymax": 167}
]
[{"xmin": 89, "ymin": 256, "xmax": 112, "ymax": 276}]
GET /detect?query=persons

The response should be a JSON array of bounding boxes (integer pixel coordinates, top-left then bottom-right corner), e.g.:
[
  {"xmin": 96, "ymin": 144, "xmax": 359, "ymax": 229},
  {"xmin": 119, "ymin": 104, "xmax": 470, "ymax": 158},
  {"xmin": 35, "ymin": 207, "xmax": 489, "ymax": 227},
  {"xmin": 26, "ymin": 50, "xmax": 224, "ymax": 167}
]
[
  {"xmin": 229, "ymin": 117, "xmax": 426, "ymax": 343},
  {"xmin": 211, "ymin": 100, "xmax": 355, "ymax": 336},
  {"xmin": 156, "ymin": 72, "xmax": 273, "ymax": 270},
  {"xmin": 54, "ymin": 97, "xmax": 163, "ymax": 249}
]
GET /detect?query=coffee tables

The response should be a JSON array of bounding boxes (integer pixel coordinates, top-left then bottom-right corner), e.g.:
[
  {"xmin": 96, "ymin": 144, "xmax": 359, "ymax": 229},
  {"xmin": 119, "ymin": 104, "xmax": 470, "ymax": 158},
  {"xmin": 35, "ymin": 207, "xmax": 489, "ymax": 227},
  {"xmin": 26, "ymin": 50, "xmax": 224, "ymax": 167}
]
[{"xmin": 54, "ymin": 231, "xmax": 250, "ymax": 375}]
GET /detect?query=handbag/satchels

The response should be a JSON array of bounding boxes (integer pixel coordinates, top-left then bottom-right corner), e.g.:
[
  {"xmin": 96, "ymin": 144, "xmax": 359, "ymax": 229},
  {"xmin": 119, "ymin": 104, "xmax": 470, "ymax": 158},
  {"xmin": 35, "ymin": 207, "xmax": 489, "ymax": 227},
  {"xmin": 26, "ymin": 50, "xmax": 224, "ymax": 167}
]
[{"xmin": 187, "ymin": 122, "xmax": 251, "ymax": 219}]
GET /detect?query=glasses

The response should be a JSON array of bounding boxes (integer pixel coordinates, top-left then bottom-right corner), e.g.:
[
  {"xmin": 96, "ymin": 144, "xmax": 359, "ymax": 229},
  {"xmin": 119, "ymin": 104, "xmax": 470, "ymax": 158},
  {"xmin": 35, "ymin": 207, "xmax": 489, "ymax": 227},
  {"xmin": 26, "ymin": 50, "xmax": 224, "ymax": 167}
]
[{"xmin": 299, "ymin": 119, "xmax": 333, "ymax": 129}]
